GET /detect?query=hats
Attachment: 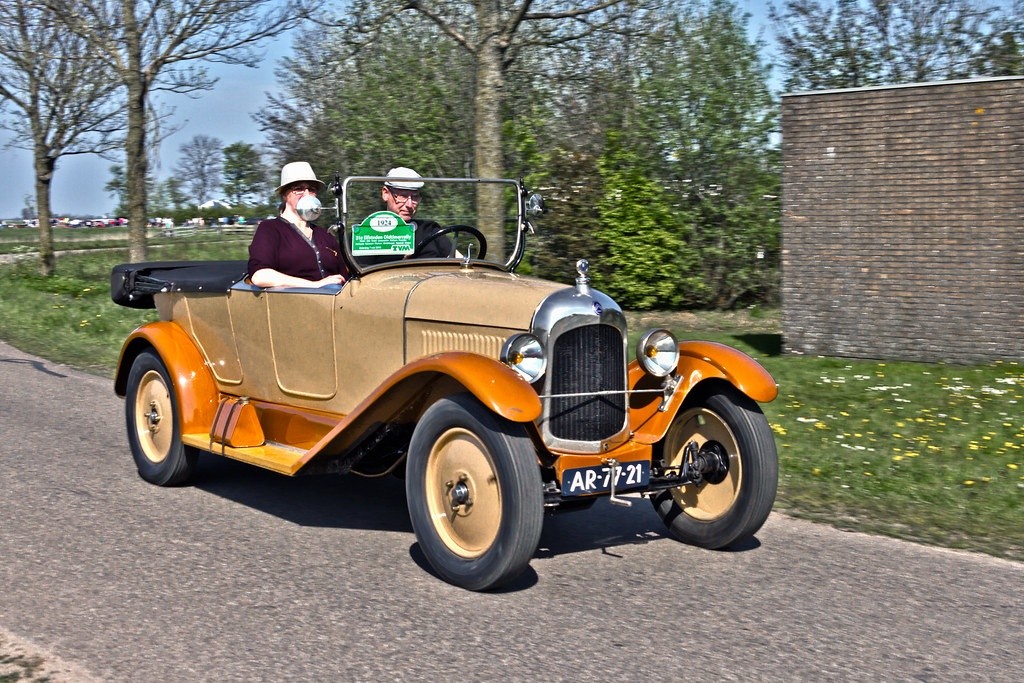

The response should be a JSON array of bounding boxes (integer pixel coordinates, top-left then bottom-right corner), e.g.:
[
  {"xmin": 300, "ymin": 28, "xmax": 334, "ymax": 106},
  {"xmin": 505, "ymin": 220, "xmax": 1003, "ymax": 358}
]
[
  {"xmin": 384, "ymin": 167, "xmax": 425, "ymax": 191},
  {"xmin": 274, "ymin": 161, "xmax": 325, "ymax": 192}
]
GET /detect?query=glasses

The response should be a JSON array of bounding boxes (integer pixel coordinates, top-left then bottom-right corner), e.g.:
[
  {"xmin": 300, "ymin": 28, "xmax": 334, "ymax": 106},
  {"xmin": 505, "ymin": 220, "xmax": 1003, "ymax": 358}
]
[
  {"xmin": 291, "ymin": 185, "xmax": 318, "ymax": 194},
  {"xmin": 389, "ymin": 192, "xmax": 422, "ymax": 204}
]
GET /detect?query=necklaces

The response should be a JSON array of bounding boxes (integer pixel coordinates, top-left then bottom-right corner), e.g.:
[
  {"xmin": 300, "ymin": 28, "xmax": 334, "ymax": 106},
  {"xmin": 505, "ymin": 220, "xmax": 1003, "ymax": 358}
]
[{"xmin": 307, "ymin": 232, "xmax": 311, "ymax": 239}]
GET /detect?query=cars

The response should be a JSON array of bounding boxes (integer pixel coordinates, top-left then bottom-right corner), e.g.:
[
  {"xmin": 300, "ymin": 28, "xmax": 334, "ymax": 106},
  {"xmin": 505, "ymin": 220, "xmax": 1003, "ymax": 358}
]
[{"xmin": 110, "ymin": 177, "xmax": 798, "ymax": 599}]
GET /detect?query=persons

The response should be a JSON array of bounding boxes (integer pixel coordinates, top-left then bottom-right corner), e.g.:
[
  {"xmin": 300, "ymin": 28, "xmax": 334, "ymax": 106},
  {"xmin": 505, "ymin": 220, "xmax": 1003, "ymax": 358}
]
[
  {"xmin": 246, "ymin": 161, "xmax": 351, "ymax": 289},
  {"xmin": 339, "ymin": 167, "xmax": 464, "ymax": 270}
]
